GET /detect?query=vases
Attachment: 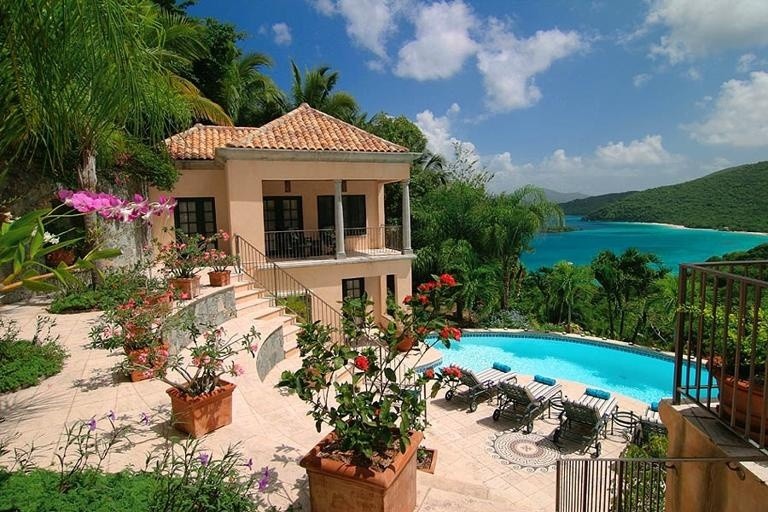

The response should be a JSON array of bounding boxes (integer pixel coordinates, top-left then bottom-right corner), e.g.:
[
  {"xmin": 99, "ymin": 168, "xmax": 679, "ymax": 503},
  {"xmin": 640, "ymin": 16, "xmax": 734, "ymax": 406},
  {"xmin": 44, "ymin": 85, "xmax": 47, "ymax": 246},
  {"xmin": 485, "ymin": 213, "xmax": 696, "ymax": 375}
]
[
  {"xmin": 208, "ymin": 270, "xmax": 231, "ymax": 286},
  {"xmin": 415, "ymin": 447, "xmax": 438, "ymax": 475},
  {"xmin": 125, "ymin": 291, "xmax": 174, "ymax": 380},
  {"xmin": 164, "ymin": 276, "xmax": 201, "ymax": 298},
  {"xmin": 299, "ymin": 422, "xmax": 423, "ymax": 511},
  {"xmin": 166, "ymin": 377, "xmax": 236, "ymax": 437}
]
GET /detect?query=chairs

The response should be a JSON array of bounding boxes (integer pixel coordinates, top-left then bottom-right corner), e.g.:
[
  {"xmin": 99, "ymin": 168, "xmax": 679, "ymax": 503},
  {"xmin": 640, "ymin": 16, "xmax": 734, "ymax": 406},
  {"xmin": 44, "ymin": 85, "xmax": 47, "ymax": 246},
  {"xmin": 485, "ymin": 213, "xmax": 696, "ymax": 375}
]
[
  {"xmin": 444, "ymin": 362, "xmax": 519, "ymax": 411},
  {"xmin": 555, "ymin": 388, "xmax": 616, "ymax": 457},
  {"xmin": 288, "ymin": 231, "xmax": 313, "ymax": 258},
  {"xmin": 492, "ymin": 373, "xmax": 562, "ymax": 435},
  {"xmin": 636, "ymin": 400, "xmax": 670, "ymax": 454}
]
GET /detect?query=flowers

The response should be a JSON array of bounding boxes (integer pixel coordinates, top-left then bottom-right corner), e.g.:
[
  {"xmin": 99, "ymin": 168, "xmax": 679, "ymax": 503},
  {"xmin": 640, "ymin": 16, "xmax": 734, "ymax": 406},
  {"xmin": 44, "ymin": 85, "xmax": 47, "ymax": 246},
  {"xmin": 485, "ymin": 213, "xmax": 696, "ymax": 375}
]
[
  {"xmin": 148, "ymin": 226, "xmax": 229, "ymax": 277},
  {"xmin": 202, "ymin": 249, "xmax": 241, "ymax": 272},
  {"xmin": 83, "ymin": 254, "xmax": 180, "ymax": 349},
  {"xmin": 133, "ymin": 321, "xmax": 261, "ymax": 398},
  {"xmin": 277, "ymin": 272, "xmax": 463, "ymax": 462}
]
